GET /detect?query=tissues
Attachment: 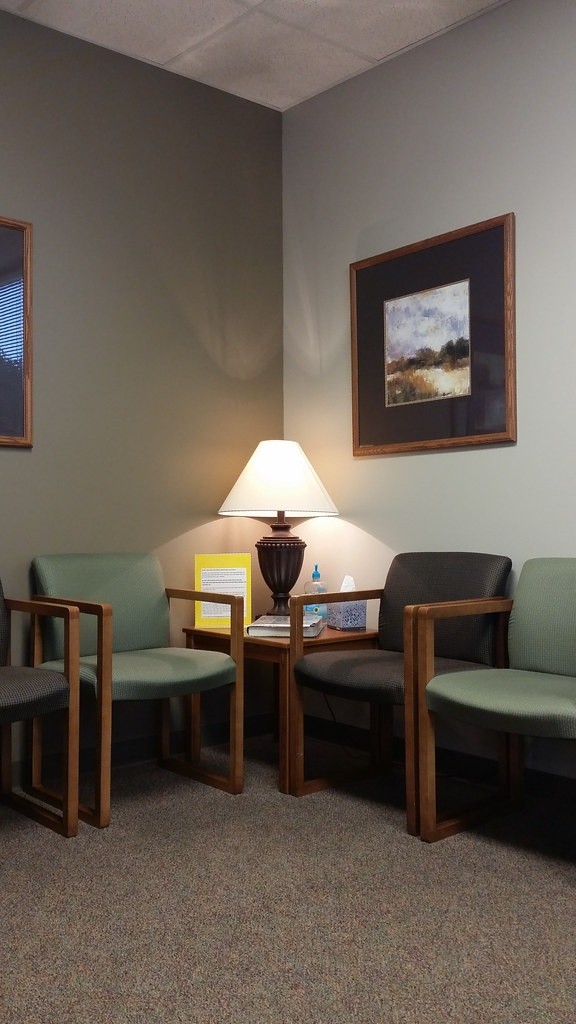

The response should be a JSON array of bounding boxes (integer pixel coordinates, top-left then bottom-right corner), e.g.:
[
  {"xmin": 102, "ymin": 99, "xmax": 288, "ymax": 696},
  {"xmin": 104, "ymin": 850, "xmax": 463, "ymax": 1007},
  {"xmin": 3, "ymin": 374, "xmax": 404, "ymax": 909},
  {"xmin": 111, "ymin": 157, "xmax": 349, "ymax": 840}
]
[{"xmin": 327, "ymin": 574, "xmax": 367, "ymax": 632}]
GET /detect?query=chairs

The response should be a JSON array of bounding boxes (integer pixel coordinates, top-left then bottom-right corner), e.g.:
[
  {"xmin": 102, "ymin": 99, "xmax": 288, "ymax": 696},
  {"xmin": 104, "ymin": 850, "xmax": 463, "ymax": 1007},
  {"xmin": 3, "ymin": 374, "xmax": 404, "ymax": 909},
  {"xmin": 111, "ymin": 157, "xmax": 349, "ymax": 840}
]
[
  {"xmin": 24, "ymin": 552, "xmax": 244, "ymax": 829},
  {"xmin": 0, "ymin": 580, "xmax": 81, "ymax": 838},
  {"xmin": 418, "ymin": 558, "xmax": 576, "ymax": 845},
  {"xmin": 288, "ymin": 550, "xmax": 512, "ymax": 837}
]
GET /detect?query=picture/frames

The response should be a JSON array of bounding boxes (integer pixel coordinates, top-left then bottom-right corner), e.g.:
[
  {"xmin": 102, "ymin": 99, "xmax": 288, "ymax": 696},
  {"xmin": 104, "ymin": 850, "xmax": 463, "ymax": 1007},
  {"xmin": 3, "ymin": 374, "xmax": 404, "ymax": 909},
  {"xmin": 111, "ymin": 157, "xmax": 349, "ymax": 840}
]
[{"xmin": 347, "ymin": 212, "xmax": 518, "ymax": 458}]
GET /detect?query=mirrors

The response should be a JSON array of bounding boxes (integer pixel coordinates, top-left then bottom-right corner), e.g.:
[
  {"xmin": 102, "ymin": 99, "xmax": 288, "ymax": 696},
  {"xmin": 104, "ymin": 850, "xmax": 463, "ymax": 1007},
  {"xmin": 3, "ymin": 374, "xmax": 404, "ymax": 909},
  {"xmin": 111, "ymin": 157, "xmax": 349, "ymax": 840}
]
[{"xmin": 0, "ymin": 216, "xmax": 34, "ymax": 449}]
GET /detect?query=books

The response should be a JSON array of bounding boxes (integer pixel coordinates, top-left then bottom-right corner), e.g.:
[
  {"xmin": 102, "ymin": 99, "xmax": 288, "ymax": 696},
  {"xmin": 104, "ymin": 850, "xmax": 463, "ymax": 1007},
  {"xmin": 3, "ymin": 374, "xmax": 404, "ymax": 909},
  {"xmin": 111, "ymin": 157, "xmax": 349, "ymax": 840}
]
[{"xmin": 247, "ymin": 614, "xmax": 325, "ymax": 638}]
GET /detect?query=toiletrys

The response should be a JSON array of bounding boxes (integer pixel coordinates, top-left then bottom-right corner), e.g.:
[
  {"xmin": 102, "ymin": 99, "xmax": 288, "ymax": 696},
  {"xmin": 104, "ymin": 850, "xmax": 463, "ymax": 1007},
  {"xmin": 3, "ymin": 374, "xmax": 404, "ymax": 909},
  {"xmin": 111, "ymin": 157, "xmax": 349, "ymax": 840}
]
[{"xmin": 304, "ymin": 563, "xmax": 328, "ymax": 628}]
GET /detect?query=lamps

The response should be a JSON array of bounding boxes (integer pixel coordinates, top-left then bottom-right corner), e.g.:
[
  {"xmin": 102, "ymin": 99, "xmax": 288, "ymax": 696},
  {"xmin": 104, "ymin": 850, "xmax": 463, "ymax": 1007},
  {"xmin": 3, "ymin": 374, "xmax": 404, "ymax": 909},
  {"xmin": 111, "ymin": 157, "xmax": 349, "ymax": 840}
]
[{"xmin": 217, "ymin": 440, "xmax": 340, "ymax": 621}]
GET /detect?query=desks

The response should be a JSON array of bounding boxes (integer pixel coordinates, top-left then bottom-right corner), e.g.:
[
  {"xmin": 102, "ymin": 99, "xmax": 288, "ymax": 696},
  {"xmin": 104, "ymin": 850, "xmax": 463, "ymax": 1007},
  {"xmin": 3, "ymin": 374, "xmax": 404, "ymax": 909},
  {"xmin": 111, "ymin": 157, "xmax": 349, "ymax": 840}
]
[{"xmin": 182, "ymin": 621, "xmax": 379, "ymax": 795}]
